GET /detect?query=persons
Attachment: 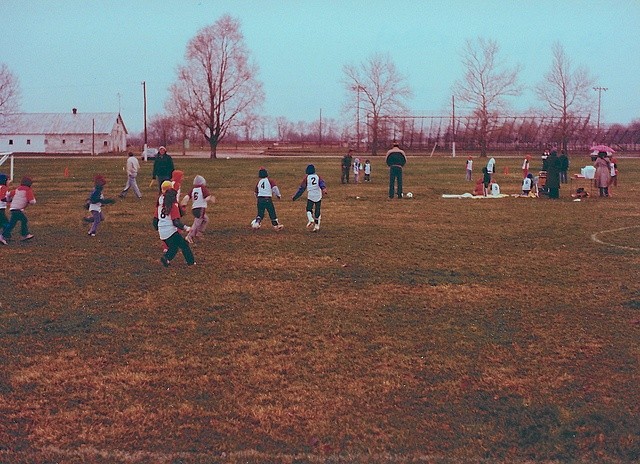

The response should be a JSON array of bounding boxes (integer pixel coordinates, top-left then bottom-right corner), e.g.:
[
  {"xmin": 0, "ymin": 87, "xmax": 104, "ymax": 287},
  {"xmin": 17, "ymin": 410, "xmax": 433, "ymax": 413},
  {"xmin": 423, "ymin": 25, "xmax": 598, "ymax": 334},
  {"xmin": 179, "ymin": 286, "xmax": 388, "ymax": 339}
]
[
  {"xmin": 363, "ymin": 160, "xmax": 372, "ymax": 182},
  {"xmin": 490, "ymin": 179, "xmax": 500, "ymax": 195},
  {"xmin": 558, "ymin": 149, "xmax": 568, "ymax": 184},
  {"xmin": 0, "ymin": 176, "xmax": 36, "ymax": 245},
  {"xmin": 0, "ymin": 175, "xmax": 16, "ymax": 241},
  {"xmin": 156, "ymin": 181, "xmax": 184, "ymax": 253},
  {"xmin": 118, "ymin": 151, "xmax": 143, "ymax": 200},
  {"xmin": 82, "ymin": 176, "xmax": 115, "ymax": 237},
  {"xmin": 293, "ymin": 164, "xmax": 327, "ymax": 233},
  {"xmin": 541, "ymin": 148, "xmax": 552, "ymax": 163},
  {"xmin": 341, "ymin": 148, "xmax": 354, "ymax": 184},
  {"xmin": 181, "ymin": 175, "xmax": 216, "ymax": 248},
  {"xmin": 473, "ymin": 178, "xmax": 484, "ymax": 195},
  {"xmin": 481, "ymin": 167, "xmax": 491, "ymax": 197},
  {"xmin": 386, "ymin": 142, "xmax": 407, "ymax": 201},
  {"xmin": 522, "ymin": 155, "xmax": 532, "ymax": 178},
  {"xmin": 253, "ymin": 166, "xmax": 284, "ymax": 232},
  {"xmin": 352, "ymin": 158, "xmax": 362, "ymax": 184},
  {"xmin": 609, "ymin": 157, "xmax": 618, "ymax": 187},
  {"xmin": 465, "ymin": 155, "xmax": 474, "ymax": 181},
  {"xmin": 543, "ymin": 147, "xmax": 562, "ymax": 199},
  {"xmin": 152, "ymin": 188, "xmax": 196, "ymax": 268},
  {"xmin": 594, "ymin": 151, "xmax": 612, "ymax": 197},
  {"xmin": 522, "ymin": 174, "xmax": 533, "ymax": 196},
  {"xmin": 171, "ymin": 170, "xmax": 185, "ymax": 217},
  {"xmin": 487, "ymin": 156, "xmax": 496, "ymax": 186},
  {"xmin": 152, "ymin": 146, "xmax": 175, "ymax": 194}
]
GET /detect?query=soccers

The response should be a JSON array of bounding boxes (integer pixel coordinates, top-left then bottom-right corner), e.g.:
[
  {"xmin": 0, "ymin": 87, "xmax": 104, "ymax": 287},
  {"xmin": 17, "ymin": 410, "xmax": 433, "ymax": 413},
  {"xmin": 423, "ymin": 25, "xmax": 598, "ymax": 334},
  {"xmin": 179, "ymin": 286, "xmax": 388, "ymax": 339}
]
[
  {"xmin": 251, "ymin": 219, "xmax": 262, "ymax": 229},
  {"xmin": 407, "ymin": 192, "xmax": 412, "ymax": 198}
]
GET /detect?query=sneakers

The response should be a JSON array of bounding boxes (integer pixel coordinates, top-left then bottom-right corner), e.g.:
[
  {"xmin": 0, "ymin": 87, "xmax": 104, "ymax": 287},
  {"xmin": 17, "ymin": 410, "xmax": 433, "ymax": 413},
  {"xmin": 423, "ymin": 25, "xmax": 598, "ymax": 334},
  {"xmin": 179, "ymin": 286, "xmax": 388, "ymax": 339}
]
[
  {"xmin": 0, "ymin": 233, "xmax": 8, "ymax": 246},
  {"xmin": 88, "ymin": 230, "xmax": 96, "ymax": 236},
  {"xmin": 312, "ymin": 225, "xmax": 320, "ymax": 232},
  {"xmin": 341, "ymin": 181, "xmax": 346, "ymax": 184},
  {"xmin": 138, "ymin": 195, "xmax": 143, "ymax": 200},
  {"xmin": 347, "ymin": 180, "xmax": 350, "ymax": 183},
  {"xmin": 251, "ymin": 219, "xmax": 262, "ymax": 231},
  {"xmin": 306, "ymin": 219, "xmax": 314, "ymax": 228},
  {"xmin": 20, "ymin": 233, "xmax": 35, "ymax": 242},
  {"xmin": 118, "ymin": 194, "xmax": 124, "ymax": 198},
  {"xmin": 161, "ymin": 255, "xmax": 171, "ymax": 266},
  {"xmin": 273, "ymin": 224, "xmax": 285, "ymax": 233},
  {"xmin": 196, "ymin": 232, "xmax": 202, "ymax": 242},
  {"xmin": 187, "ymin": 262, "xmax": 196, "ymax": 267},
  {"xmin": 186, "ymin": 234, "xmax": 194, "ymax": 244}
]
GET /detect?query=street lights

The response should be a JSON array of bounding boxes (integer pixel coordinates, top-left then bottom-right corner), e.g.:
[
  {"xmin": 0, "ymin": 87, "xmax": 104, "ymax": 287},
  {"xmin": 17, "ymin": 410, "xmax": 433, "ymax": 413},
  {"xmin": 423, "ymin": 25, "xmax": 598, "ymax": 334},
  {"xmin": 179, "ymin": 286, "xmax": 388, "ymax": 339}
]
[
  {"xmin": 352, "ymin": 85, "xmax": 367, "ymax": 148},
  {"xmin": 594, "ymin": 87, "xmax": 608, "ymax": 128}
]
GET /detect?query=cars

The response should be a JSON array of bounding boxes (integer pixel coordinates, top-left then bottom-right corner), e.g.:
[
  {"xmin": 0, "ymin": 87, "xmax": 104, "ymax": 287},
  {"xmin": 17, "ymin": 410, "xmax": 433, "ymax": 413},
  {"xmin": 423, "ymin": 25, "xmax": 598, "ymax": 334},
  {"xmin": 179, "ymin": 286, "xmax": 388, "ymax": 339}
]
[{"xmin": 142, "ymin": 149, "xmax": 158, "ymax": 159}]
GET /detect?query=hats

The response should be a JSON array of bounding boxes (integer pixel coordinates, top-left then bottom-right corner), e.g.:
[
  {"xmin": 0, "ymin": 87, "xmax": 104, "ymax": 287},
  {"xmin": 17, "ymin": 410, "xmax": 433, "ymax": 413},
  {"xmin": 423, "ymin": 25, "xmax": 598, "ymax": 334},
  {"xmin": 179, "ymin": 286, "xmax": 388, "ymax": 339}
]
[
  {"xmin": 159, "ymin": 145, "xmax": 166, "ymax": 151},
  {"xmin": 527, "ymin": 173, "xmax": 533, "ymax": 178},
  {"xmin": 161, "ymin": 181, "xmax": 172, "ymax": 190},
  {"xmin": 550, "ymin": 147, "xmax": 557, "ymax": 153},
  {"xmin": 21, "ymin": 176, "xmax": 33, "ymax": 186},
  {"xmin": 466, "ymin": 155, "xmax": 473, "ymax": 159},
  {"xmin": 305, "ymin": 164, "xmax": 315, "ymax": 174},
  {"xmin": 524, "ymin": 154, "xmax": 532, "ymax": 159},
  {"xmin": 192, "ymin": 174, "xmax": 206, "ymax": 185},
  {"xmin": 93, "ymin": 174, "xmax": 108, "ymax": 186},
  {"xmin": 258, "ymin": 167, "xmax": 268, "ymax": 178},
  {"xmin": 355, "ymin": 158, "xmax": 360, "ymax": 163}
]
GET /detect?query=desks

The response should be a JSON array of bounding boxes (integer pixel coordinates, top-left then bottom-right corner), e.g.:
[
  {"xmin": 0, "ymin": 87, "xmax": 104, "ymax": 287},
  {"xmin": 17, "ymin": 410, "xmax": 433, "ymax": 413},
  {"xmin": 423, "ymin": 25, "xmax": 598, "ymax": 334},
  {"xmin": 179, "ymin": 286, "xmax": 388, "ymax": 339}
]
[{"xmin": 570, "ymin": 176, "xmax": 599, "ymax": 197}]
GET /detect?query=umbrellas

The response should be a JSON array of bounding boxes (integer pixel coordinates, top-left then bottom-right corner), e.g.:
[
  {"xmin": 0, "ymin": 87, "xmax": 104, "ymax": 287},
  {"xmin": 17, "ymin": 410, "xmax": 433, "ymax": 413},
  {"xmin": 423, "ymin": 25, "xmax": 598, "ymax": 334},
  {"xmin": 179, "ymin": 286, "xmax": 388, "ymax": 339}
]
[{"xmin": 589, "ymin": 144, "xmax": 615, "ymax": 152}]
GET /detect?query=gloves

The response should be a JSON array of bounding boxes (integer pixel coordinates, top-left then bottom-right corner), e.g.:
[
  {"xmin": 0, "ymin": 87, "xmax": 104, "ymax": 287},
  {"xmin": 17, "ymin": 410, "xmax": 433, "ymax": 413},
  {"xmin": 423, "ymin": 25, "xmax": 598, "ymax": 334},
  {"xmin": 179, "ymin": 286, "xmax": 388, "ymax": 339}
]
[
  {"xmin": 182, "ymin": 207, "xmax": 187, "ymax": 213},
  {"xmin": 185, "ymin": 225, "xmax": 193, "ymax": 232},
  {"xmin": 277, "ymin": 194, "xmax": 281, "ymax": 199},
  {"xmin": 322, "ymin": 189, "xmax": 327, "ymax": 195},
  {"xmin": 292, "ymin": 196, "xmax": 296, "ymax": 202}
]
